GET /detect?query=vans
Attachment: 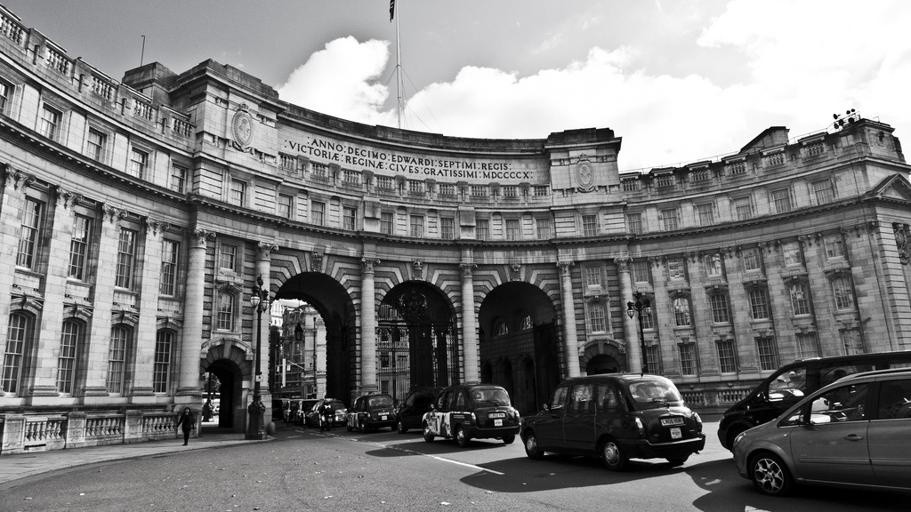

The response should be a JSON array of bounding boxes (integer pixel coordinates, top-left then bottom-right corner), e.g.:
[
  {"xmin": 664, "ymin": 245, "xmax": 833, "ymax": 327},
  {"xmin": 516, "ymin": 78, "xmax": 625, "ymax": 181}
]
[{"xmin": 715, "ymin": 349, "xmax": 910, "ymax": 458}]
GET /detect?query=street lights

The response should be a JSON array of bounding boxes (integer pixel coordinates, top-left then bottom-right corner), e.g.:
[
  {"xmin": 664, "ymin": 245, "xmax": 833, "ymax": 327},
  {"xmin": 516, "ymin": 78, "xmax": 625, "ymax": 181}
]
[
  {"xmin": 242, "ymin": 272, "xmax": 269, "ymax": 441},
  {"xmin": 626, "ymin": 289, "xmax": 653, "ymax": 375},
  {"xmin": 293, "ymin": 316, "xmax": 318, "ymax": 399}
]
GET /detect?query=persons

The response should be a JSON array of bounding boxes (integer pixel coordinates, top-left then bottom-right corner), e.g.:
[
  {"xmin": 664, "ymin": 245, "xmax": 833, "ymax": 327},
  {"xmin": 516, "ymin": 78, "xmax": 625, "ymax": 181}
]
[
  {"xmin": 204, "ymin": 399, "xmax": 215, "ymax": 418},
  {"xmin": 177, "ymin": 405, "xmax": 196, "ymax": 446},
  {"xmin": 831, "ymin": 369, "xmax": 853, "ymax": 393},
  {"xmin": 319, "ymin": 399, "xmax": 334, "ymax": 431},
  {"xmin": 287, "ymin": 403, "xmax": 300, "ymax": 421}
]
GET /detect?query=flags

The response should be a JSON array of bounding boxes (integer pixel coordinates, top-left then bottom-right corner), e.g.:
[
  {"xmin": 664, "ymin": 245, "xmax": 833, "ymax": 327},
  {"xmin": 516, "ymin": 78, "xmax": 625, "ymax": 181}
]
[{"xmin": 389, "ymin": 0, "xmax": 395, "ymax": 23}]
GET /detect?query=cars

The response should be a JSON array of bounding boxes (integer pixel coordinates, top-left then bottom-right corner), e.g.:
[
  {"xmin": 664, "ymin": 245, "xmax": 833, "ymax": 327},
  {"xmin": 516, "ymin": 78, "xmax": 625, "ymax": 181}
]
[
  {"xmin": 728, "ymin": 366, "xmax": 910, "ymax": 502},
  {"xmin": 419, "ymin": 381, "xmax": 521, "ymax": 446},
  {"xmin": 519, "ymin": 371, "xmax": 707, "ymax": 473},
  {"xmin": 269, "ymin": 382, "xmax": 448, "ymax": 436},
  {"xmin": 210, "ymin": 401, "xmax": 220, "ymax": 415}
]
